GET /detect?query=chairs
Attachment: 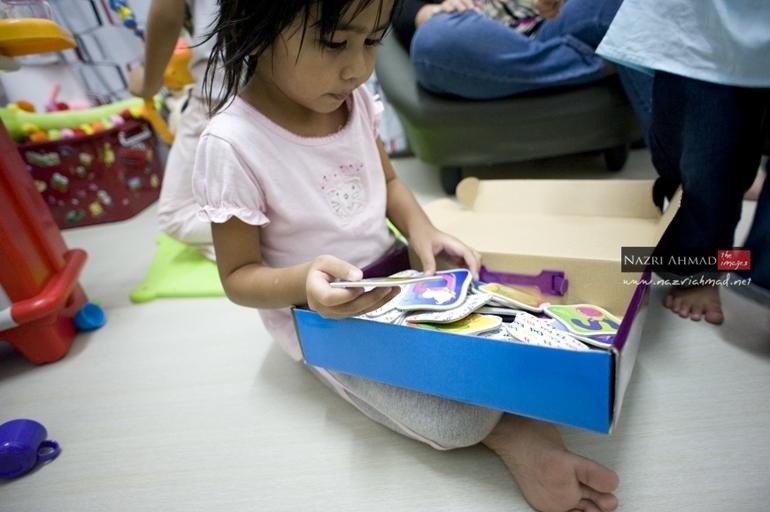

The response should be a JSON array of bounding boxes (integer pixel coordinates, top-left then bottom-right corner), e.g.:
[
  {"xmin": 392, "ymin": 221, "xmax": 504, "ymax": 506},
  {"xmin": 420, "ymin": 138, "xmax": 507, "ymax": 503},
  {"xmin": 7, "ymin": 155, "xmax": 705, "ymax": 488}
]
[{"xmin": 368, "ymin": 27, "xmax": 629, "ymax": 195}]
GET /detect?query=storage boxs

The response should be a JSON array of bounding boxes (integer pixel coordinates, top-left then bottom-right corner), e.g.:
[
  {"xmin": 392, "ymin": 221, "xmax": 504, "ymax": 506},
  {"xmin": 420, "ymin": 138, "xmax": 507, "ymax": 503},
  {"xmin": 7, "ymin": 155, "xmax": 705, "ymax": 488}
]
[
  {"xmin": 291, "ymin": 177, "xmax": 685, "ymax": 432},
  {"xmin": 16, "ymin": 112, "xmax": 163, "ymax": 233}
]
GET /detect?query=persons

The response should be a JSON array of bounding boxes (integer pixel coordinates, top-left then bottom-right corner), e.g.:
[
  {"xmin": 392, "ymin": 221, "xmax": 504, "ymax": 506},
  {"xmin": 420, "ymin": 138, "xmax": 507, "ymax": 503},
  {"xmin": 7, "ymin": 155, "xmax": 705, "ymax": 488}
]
[
  {"xmin": 594, "ymin": 1, "xmax": 770, "ymax": 325},
  {"xmin": 0, "ymin": 99, "xmax": 177, "ymax": 229},
  {"xmin": 391, "ymin": 0, "xmax": 767, "ymax": 201},
  {"xmin": 180, "ymin": 1, "xmax": 621, "ymax": 512},
  {"xmin": 127, "ymin": 1, "xmax": 250, "ymax": 264}
]
